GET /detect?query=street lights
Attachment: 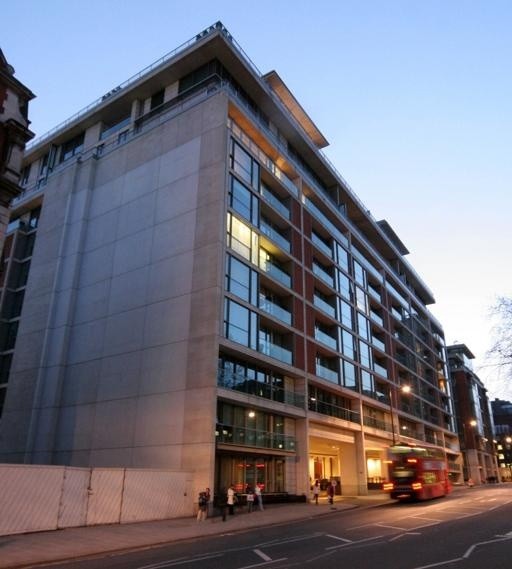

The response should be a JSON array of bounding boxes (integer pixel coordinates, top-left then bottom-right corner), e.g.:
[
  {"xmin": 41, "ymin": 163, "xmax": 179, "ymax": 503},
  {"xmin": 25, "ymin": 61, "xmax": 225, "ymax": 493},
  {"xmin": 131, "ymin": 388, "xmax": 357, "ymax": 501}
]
[
  {"xmin": 503, "ymin": 435, "xmax": 512, "ymax": 467},
  {"xmin": 462, "ymin": 420, "xmax": 478, "ymax": 485},
  {"xmin": 388, "ymin": 385, "xmax": 416, "ymax": 446}
]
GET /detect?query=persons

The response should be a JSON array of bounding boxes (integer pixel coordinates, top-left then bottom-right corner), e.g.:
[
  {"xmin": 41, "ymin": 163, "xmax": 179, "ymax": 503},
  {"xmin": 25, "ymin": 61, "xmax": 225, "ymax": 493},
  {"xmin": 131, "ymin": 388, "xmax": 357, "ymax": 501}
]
[
  {"xmin": 468, "ymin": 477, "xmax": 472, "ymax": 487},
  {"xmin": 481, "ymin": 476, "xmax": 505, "ymax": 484},
  {"xmin": 247, "ymin": 490, "xmax": 254, "ymax": 513},
  {"xmin": 326, "ymin": 480, "xmax": 337, "ymax": 505},
  {"xmin": 226, "ymin": 484, "xmax": 237, "ymax": 515},
  {"xmin": 312, "ymin": 480, "xmax": 321, "ymax": 504},
  {"xmin": 196, "ymin": 488, "xmax": 210, "ymax": 523},
  {"xmin": 255, "ymin": 480, "xmax": 265, "ymax": 512}
]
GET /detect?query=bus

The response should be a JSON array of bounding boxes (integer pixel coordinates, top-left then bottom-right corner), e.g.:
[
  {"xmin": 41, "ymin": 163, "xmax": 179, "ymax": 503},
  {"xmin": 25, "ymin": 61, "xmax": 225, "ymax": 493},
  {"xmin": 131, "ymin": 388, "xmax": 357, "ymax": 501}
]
[
  {"xmin": 384, "ymin": 443, "xmax": 451, "ymax": 503},
  {"xmin": 237, "ymin": 463, "xmax": 269, "ymax": 494}
]
